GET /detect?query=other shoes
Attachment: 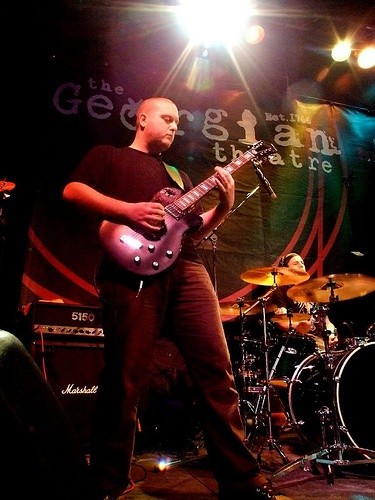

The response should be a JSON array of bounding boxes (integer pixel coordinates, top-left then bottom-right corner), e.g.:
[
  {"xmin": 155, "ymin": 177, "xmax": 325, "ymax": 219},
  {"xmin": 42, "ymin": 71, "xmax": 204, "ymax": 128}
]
[
  {"xmin": 244, "ymin": 482, "xmax": 276, "ymax": 500},
  {"xmin": 99, "ymin": 493, "xmax": 110, "ymax": 499}
]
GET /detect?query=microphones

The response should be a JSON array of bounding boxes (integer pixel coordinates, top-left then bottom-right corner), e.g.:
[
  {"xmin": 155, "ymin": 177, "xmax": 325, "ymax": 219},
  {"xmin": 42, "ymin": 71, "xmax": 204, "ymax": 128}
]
[{"xmin": 252, "ymin": 160, "xmax": 277, "ymax": 201}]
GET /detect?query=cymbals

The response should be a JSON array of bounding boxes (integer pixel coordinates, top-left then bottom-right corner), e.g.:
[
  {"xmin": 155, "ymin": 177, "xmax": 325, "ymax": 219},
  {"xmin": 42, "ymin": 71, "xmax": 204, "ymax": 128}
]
[
  {"xmin": 218, "ymin": 300, "xmax": 278, "ymax": 315},
  {"xmin": 240, "ymin": 266, "xmax": 311, "ymax": 286},
  {"xmin": 271, "ymin": 313, "xmax": 311, "ymax": 322},
  {"xmin": 287, "ymin": 274, "xmax": 375, "ymax": 303}
]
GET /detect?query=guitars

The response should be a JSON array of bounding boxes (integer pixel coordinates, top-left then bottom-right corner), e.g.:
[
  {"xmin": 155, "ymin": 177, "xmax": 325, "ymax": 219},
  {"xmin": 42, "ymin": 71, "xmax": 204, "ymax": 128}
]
[{"xmin": 99, "ymin": 140, "xmax": 278, "ymax": 276}]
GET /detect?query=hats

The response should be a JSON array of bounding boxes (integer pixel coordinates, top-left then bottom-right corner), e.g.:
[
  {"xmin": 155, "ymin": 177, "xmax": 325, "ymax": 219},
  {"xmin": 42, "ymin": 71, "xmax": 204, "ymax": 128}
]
[{"xmin": 278, "ymin": 253, "xmax": 299, "ymax": 267}]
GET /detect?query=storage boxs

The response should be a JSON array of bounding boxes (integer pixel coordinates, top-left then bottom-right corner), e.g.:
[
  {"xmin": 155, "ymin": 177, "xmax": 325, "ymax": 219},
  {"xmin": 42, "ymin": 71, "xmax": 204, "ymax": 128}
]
[{"xmin": 32, "ymin": 340, "xmax": 106, "ymax": 425}]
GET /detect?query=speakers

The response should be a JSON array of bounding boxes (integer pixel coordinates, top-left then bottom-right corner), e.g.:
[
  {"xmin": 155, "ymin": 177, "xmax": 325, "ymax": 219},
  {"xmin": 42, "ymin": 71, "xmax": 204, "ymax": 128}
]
[{"xmin": 28, "ymin": 340, "xmax": 106, "ymax": 432}]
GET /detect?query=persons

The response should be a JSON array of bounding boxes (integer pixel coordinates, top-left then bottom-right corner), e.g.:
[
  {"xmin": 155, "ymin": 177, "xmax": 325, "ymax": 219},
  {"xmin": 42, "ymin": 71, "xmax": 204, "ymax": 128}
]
[
  {"xmin": 61, "ymin": 97, "xmax": 276, "ymax": 500},
  {"xmin": 279, "ymin": 252, "xmax": 340, "ymax": 351}
]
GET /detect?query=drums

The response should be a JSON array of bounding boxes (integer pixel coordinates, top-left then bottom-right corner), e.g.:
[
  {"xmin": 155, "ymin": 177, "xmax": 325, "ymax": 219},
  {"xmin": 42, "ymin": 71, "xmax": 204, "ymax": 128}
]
[
  {"xmin": 226, "ymin": 336, "xmax": 267, "ymax": 393},
  {"xmin": 267, "ymin": 333, "xmax": 317, "ymax": 387},
  {"xmin": 289, "ymin": 341, "xmax": 375, "ymax": 474}
]
